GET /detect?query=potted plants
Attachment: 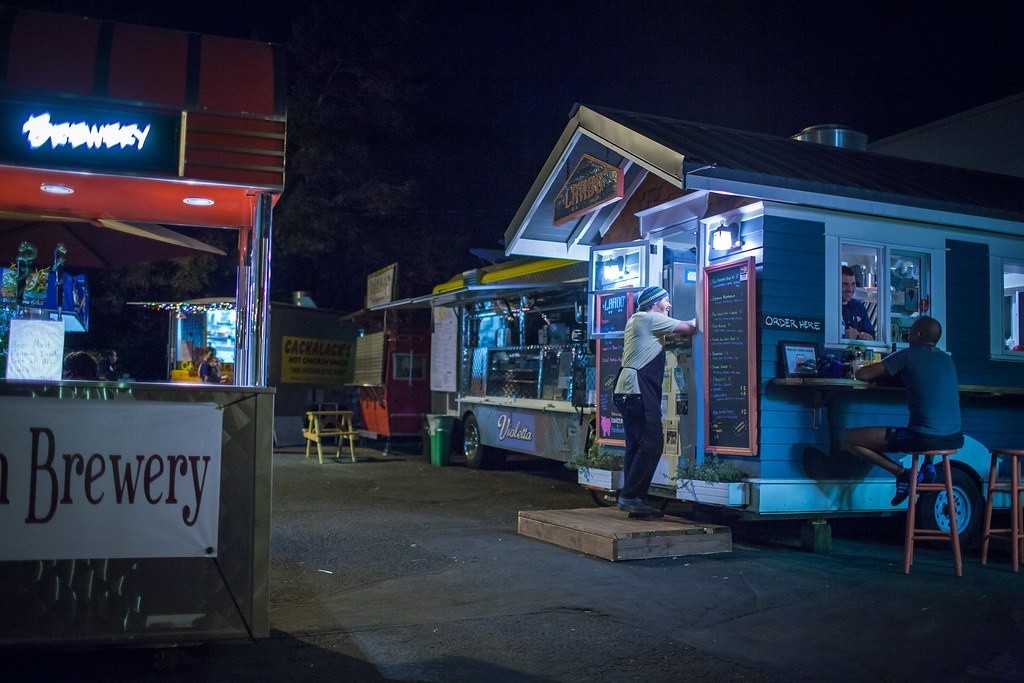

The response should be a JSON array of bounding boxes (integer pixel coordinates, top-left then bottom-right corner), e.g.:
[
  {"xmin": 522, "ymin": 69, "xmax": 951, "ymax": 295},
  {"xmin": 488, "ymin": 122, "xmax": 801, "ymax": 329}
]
[
  {"xmin": 661, "ymin": 456, "xmax": 751, "ymax": 511},
  {"xmin": 563, "ymin": 438, "xmax": 624, "ymax": 490}
]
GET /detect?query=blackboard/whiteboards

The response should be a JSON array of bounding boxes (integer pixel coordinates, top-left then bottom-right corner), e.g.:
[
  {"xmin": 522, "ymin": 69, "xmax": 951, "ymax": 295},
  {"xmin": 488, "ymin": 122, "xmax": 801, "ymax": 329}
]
[
  {"xmin": 6, "ymin": 319, "xmax": 65, "ymax": 381},
  {"xmin": 595, "ymin": 284, "xmax": 633, "ymax": 448},
  {"xmin": 702, "ymin": 255, "xmax": 758, "ymax": 457}
]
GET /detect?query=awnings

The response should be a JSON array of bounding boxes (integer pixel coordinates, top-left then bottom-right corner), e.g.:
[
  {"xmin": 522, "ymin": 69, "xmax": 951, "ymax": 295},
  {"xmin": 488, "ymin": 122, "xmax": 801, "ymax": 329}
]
[{"xmin": 339, "ymin": 283, "xmax": 576, "ymax": 322}]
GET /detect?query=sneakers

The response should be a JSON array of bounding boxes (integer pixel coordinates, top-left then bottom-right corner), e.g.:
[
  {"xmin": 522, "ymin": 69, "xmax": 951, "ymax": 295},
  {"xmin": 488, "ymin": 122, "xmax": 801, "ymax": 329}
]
[
  {"xmin": 916, "ymin": 462, "xmax": 936, "ymax": 495},
  {"xmin": 891, "ymin": 468, "xmax": 918, "ymax": 506}
]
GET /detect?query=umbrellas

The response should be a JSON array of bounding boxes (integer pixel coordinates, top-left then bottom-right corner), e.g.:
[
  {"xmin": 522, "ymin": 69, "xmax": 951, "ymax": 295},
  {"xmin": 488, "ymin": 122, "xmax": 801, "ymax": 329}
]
[{"xmin": 0, "ymin": 210, "xmax": 227, "ymax": 270}]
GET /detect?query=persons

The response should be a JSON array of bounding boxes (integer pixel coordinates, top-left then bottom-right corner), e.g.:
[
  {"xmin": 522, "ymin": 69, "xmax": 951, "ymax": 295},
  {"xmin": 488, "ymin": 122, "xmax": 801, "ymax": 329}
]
[
  {"xmin": 613, "ymin": 287, "xmax": 696, "ymax": 519},
  {"xmin": 62, "ymin": 351, "xmax": 115, "ymax": 399},
  {"xmin": 840, "ymin": 316, "xmax": 964, "ymax": 506},
  {"xmin": 100, "ymin": 349, "xmax": 118, "ymax": 381},
  {"xmin": 198, "ymin": 347, "xmax": 233, "ymax": 384},
  {"xmin": 842, "ymin": 265, "xmax": 876, "ymax": 341}
]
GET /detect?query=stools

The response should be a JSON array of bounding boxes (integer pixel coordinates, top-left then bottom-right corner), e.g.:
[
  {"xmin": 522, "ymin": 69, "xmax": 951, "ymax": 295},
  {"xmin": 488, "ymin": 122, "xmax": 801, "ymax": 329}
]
[
  {"xmin": 980, "ymin": 447, "xmax": 1024, "ymax": 573},
  {"xmin": 903, "ymin": 449, "xmax": 963, "ymax": 576}
]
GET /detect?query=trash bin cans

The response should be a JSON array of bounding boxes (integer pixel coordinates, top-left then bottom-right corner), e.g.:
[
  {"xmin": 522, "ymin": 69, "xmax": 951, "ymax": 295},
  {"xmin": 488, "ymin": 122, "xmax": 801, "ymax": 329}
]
[{"xmin": 419, "ymin": 413, "xmax": 454, "ymax": 467}]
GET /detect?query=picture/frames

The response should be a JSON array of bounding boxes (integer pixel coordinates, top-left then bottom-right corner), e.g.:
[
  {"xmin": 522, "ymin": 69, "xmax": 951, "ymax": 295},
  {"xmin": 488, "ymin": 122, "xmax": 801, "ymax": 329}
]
[{"xmin": 777, "ymin": 338, "xmax": 823, "ymax": 378}]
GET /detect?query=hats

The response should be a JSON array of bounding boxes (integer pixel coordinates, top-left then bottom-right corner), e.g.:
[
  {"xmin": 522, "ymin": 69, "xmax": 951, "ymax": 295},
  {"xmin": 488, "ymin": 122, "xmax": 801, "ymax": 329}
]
[
  {"xmin": 637, "ymin": 286, "xmax": 669, "ymax": 311},
  {"xmin": 64, "ymin": 351, "xmax": 103, "ymax": 375}
]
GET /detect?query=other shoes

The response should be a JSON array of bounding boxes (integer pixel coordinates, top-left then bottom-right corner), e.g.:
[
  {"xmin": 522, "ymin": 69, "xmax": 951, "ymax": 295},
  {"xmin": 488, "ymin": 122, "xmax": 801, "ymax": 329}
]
[
  {"xmin": 617, "ymin": 497, "xmax": 654, "ymax": 515},
  {"xmin": 628, "ymin": 499, "xmax": 665, "ymax": 519}
]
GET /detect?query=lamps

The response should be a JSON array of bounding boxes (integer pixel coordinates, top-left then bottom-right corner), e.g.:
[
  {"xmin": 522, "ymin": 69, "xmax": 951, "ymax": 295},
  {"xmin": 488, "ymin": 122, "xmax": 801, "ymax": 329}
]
[
  {"xmin": 712, "ymin": 220, "xmax": 739, "ymax": 251},
  {"xmin": 602, "ymin": 255, "xmax": 624, "ymax": 280}
]
